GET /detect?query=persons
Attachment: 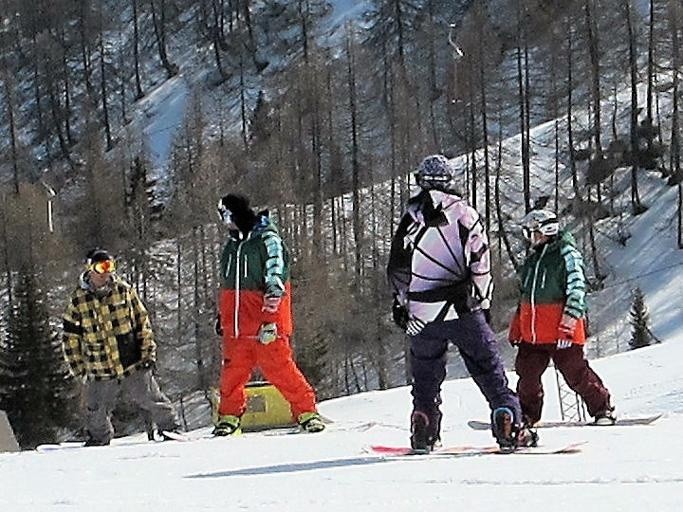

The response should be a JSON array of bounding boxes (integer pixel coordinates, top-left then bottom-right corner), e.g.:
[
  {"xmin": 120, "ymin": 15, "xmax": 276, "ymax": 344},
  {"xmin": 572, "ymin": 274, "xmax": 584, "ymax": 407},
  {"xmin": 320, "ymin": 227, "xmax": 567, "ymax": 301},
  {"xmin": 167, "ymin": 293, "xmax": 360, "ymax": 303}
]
[
  {"xmin": 210, "ymin": 193, "xmax": 325, "ymax": 437},
  {"xmin": 386, "ymin": 156, "xmax": 539, "ymax": 453},
  {"xmin": 510, "ymin": 209, "xmax": 616, "ymax": 428},
  {"xmin": 61, "ymin": 249, "xmax": 180, "ymax": 448}
]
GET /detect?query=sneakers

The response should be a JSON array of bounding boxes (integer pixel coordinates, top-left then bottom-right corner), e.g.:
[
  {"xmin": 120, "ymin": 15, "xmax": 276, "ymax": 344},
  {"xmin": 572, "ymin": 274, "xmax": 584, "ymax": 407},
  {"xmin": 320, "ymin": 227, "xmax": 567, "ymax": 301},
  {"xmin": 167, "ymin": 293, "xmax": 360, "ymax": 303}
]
[
  {"xmin": 298, "ymin": 413, "xmax": 325, "ymax": 431},
  {"xmin": 163, "ymin": 426, "xmax": 184, "ymax": 441},
  {"xmin": 409, "ymin": 435, "xmax": 444, "ymax": 454},
  {"xmin": 82, "ymin": 435, "xmax": 112, "ymax": 446},
  {"xmin": 595, "ymin": 398, "xmax": 615, "ymax": 426},
  {"xmin": 214, "ymin": 415, "xmax": 241, "ymax": 437},
  {"xmin": 492, "ymin": 429, "xmax": 539, "ymax": 451}
]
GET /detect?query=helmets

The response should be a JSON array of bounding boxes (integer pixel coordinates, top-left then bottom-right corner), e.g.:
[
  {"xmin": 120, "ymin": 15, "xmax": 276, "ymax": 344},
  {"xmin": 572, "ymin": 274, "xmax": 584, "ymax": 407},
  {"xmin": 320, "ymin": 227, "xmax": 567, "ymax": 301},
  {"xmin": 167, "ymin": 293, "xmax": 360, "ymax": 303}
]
[
  {"xmin": 416, "ymin": 155, "xmax": 452, "ymax": 186},
  {"xmin": 522, "ymin": 209, "xmax": 558, "ymax": 242},
  {"xmin": 87, "ymin": 250, "xmax": 115, "ymax": 275},
  {"xmin": 217, "ymin": 193, "xmax": 250, "ymax": 222}
]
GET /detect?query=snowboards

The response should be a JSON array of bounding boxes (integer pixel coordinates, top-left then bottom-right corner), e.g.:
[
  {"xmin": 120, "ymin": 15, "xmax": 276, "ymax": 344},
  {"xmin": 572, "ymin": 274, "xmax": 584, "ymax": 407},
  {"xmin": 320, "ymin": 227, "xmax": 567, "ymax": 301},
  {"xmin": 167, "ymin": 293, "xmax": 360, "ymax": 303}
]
[
  {"xmin": 373, "ymin": 446, "xmax": 584, "ymax": 456},
  {"xmin": 162, "ymin": 423, "xmax": 379, "ymax": 443},
  {"xmin": 36, "ymin": 440, "xmax": 176, "ymax": 452},
  {"xmin": 468, "ymin": 419, "xmax": 651, "ymax": 429}
]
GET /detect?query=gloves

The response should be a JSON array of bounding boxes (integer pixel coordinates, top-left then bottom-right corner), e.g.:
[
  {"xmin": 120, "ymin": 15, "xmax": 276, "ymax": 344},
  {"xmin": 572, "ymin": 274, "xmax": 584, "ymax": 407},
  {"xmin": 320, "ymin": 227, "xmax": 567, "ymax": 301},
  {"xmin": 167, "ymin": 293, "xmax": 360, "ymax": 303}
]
[
  {"xmin": 393, "ymin": 305, "xmax": 412, "ymax": 331},
  {"xmin": 213, "ymin": 315, "xmax": 224, "ymax": 336},
  {"xmin": 140, "ymin": 357, "xmax": 158, "ymax": 373},
  {"xmin": 556, "ymin": 323, "xmax": 575, "ymax": 349},
  {"xmin": 257, "ymin": 321, "xmax": 279, "ymax": 345}
]
